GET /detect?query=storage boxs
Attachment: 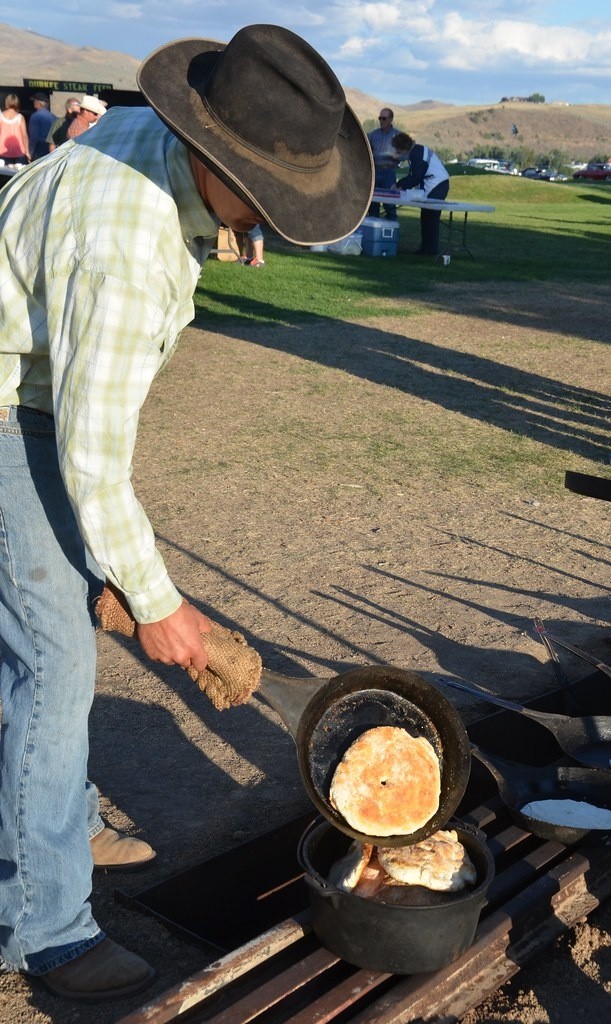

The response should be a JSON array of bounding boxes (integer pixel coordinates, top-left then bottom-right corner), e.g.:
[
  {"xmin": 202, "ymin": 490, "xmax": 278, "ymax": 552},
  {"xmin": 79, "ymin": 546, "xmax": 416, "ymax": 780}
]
[{"xmin": 312, "ymin": 217, "xmax": 402, "ymax": 255}]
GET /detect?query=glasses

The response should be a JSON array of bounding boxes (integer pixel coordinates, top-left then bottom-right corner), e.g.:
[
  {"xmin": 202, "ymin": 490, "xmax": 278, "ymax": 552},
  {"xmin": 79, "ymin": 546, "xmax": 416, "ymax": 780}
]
[{"xmin": 378, "ymin": 116, "xmax": 391, "ymax": 121}]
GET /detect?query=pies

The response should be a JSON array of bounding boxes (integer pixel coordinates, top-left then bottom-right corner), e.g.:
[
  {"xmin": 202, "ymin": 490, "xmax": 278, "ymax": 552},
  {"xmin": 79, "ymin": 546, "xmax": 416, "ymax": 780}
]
[
  {"xmin": 519, "ymin": 799, "xmax": 611, "ymax": 829},
  {"xmin": 328, "ymin": 727, "xmax": 441, "ymax": 837},
  {"xmin": 326, "ymin": 831, "xmax": 477, "ymax": 907}
]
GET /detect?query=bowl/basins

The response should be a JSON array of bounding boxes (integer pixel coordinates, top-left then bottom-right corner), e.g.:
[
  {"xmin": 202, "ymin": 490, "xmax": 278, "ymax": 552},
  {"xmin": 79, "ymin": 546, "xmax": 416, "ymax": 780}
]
[
  {"xmin": 15, "ymin": 163, "xmax": 23, "ymax": 169},
  {"xmin": 8, "ymin": 164, "xmax": 15, "ymax": 168},
  {"xmin": 400, "ymin": 189, "xmax": 424, "ymax": 199}
]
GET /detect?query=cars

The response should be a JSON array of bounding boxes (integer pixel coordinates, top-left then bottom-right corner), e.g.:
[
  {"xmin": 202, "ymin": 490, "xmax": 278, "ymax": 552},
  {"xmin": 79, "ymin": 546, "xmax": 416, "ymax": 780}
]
[
  {"xmin": 572, "ymin": 164, "xmax": 611, "ymax": 180},
  {"xmin": 466, "ymin": 158, "xmax": 567, "ymax": 182}
]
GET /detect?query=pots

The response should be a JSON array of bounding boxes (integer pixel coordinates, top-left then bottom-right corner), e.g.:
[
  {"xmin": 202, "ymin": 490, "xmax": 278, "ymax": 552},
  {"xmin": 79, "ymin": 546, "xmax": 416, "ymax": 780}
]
[
  {"xmin": 435, "ymin": 677, "xmax": 611, "ymax": 770},
  {"xmin": 257, "ymin": 666, "xmax": 473, "ymax": 848},
  {"xmin": 468, "ymin": 740, "xmax": 611, "ymax": 842},
  {"xmin": 297, "ymin": 813, "xmax": 493, "ymax": 974}
]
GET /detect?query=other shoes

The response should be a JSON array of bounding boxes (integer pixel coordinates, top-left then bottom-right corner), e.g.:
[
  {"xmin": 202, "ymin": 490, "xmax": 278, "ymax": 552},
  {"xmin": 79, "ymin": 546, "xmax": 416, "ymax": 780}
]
[
  {"xmin": 28, "ymin": 935, "xmax": 155, "ymax": 1002},
  {"xmin": 249, "ymin": 259, "xmax": 264, "ymax": 267},
  {"xmin": 413, "ymin": 248, "xmax": 440, "ymax": 256},
  {"xmin": 235, "ymin": 256, "xmax": 254, "ymax": 265},
  {"xmin": 86, "ymin": 827, "xmax": 157, "ymax": 873}
]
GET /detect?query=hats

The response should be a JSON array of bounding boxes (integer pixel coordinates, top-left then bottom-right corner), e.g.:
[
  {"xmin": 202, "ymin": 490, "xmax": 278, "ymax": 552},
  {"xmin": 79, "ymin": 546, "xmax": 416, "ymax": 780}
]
[
  {"xmin": 136, "ymin": 24, "xmax": 375, "ymax": 247},
  {"xmin": 76, "ymin": 95, "xmax": 108, "ymax": 117},
  {"xmin": 30, "ymin": 93, "xmax": 49, "ymax": 103}
]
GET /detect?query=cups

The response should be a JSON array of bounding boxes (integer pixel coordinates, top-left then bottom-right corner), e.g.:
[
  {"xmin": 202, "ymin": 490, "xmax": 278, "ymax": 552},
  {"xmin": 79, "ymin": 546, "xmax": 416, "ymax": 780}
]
[
  {"xmin": 0, "ymin": 159, "xmax": 4, "ymax": 167},
  {"xmin": 442, "ymin": 254, "xmax": 450, "ymax": 265}
]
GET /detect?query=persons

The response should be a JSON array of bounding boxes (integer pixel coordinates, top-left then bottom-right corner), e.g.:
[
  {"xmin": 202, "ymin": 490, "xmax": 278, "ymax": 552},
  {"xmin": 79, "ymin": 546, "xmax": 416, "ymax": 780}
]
[
  {"xmin": 391, "ymin": 132, "xmax": 450, "ymax": 255},
  {"xmin": 238, "ymin": 224, "xmax": 265, "ymax": 266},
  {"xmin": 28, "ymin": 92, "xmax": 58, "ymax": 166},
  {"xmin": 0, "ymin": 94, "xmax": 32, "ymax": 166},
  {"xmin": 66, "ymin": 95, "xmax": 107, "ymax": 141},
  {"xmin": 0, "ymin": 25, "xmax": 374, "ymax": 998},
  {"xmin": 45, "ymin": 98, "xmax": 81, "ymax": 152},
  {"xmin": 367, "ymin": 108, "xmax": 411, "ymax": 220}
]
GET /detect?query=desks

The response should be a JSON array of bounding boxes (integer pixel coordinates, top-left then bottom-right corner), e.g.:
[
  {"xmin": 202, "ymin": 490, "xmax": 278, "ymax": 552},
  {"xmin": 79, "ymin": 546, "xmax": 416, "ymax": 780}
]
[{"xmin": 370, "ymin": 192, "xmax": 495, "ymax": 261}]
